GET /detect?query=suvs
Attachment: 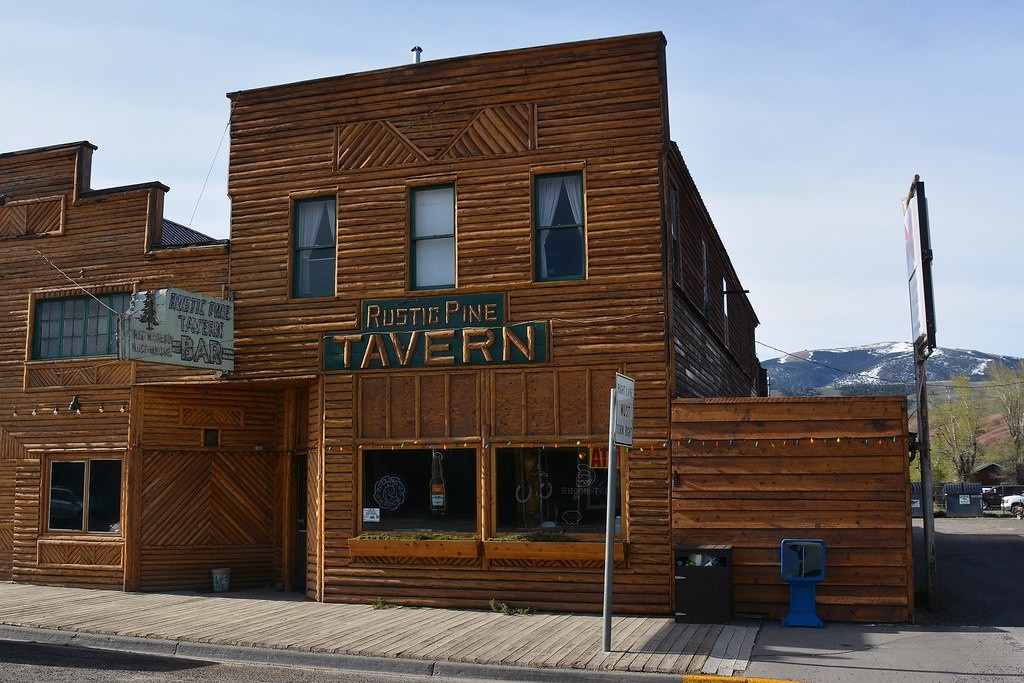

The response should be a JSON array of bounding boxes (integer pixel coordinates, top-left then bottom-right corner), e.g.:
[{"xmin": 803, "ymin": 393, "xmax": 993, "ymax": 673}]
[
  {"xmin": 1001, "ymin": 491, "xmax": 1024, "ymax": 519},
  {"xmin": 982, "ymin": 485, "xmax": 1024, "ymax": 510}
]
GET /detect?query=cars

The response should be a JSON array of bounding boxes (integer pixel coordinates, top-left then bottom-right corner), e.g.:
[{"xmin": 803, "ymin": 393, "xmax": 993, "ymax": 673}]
[{"xmin": 50, "ymin": 486, "xmax": 83, "ymax": 525}]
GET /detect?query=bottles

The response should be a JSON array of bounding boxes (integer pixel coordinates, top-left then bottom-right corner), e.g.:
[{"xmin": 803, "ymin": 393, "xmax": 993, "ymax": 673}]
[{"xmin": 429, "ymin": 455, "xmax": 447, "ymax": 514}]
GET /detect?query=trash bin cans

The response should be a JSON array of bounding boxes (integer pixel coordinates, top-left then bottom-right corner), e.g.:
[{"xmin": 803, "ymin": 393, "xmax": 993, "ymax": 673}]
[
  {"xmin": 673, "ymin": 545, "xmax": 734, "ymax": 624},
  {"xmin": 212, "ymin": 569, "xmax": 230, "ymax": 592},
  {"xmin": 943, "ymin": 483, "xmax": 983, "ymax": 518},
  {"xmin": 910, "ymin": 482, "xmax": 923, "ymax": 517}
]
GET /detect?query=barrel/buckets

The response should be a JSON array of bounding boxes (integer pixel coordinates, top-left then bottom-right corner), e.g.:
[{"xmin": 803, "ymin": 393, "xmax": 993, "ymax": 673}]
[{"xmin": 211, "ymin": 567, "xmax": 230, "ymax": 592}]
